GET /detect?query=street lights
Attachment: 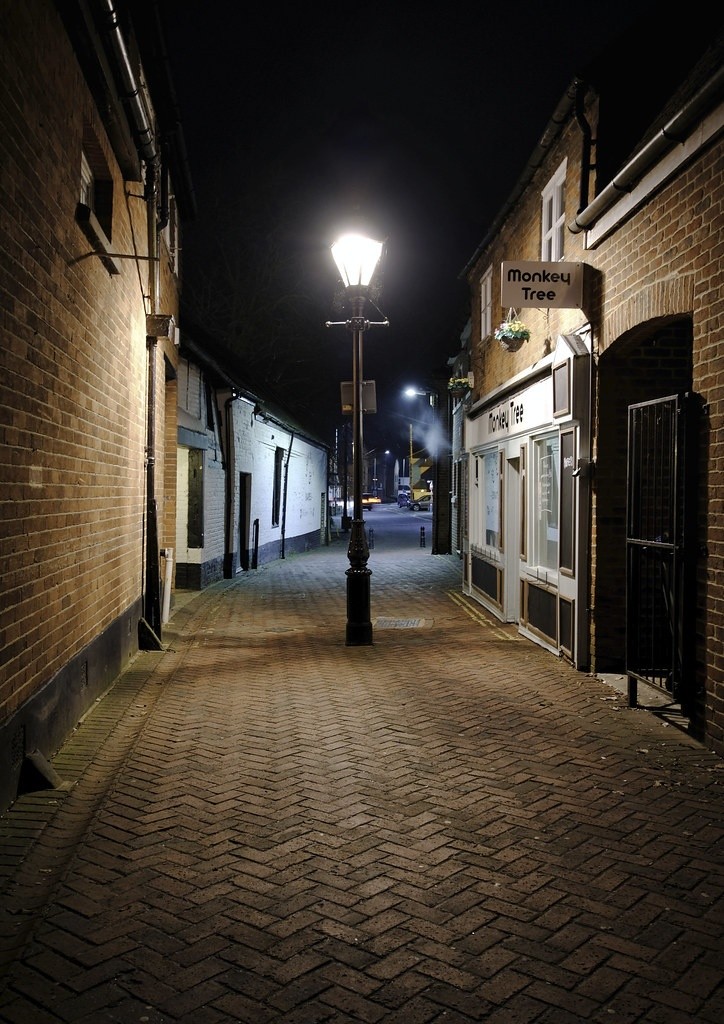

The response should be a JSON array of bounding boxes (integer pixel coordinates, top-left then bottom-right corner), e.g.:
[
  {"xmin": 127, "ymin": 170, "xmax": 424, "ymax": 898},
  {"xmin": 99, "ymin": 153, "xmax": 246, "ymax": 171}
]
[{"xmin": 327, "ymin": 231, "xmax": 391, "ymax": 647}]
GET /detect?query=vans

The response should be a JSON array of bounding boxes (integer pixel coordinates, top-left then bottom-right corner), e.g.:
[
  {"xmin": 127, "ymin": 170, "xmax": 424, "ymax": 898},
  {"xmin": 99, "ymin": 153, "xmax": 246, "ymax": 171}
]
[{"xmin": 397, "ymin": 493, "xmax": 411, "ymax": 508}]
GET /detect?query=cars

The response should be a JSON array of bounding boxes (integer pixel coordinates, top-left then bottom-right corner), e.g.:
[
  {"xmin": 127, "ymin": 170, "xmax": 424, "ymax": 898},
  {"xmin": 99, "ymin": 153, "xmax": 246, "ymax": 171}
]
[
  {"xmin": 361, "ymin": 493, "xmax": 375, "ymax": 511},
  {"xmin": 406, "ymin": 495, "xmax": 431, "ymax": 512}
]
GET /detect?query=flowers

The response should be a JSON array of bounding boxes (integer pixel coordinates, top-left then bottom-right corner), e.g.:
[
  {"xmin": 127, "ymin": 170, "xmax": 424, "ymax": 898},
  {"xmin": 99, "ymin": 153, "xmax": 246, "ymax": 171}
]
[
  {"xmin": 445, "ymin": 376, "xmax": 474, "ymax": 391},
  {"xmin": 493, "ymin": 319, "xmax": 531, "ymax": 343}
]
